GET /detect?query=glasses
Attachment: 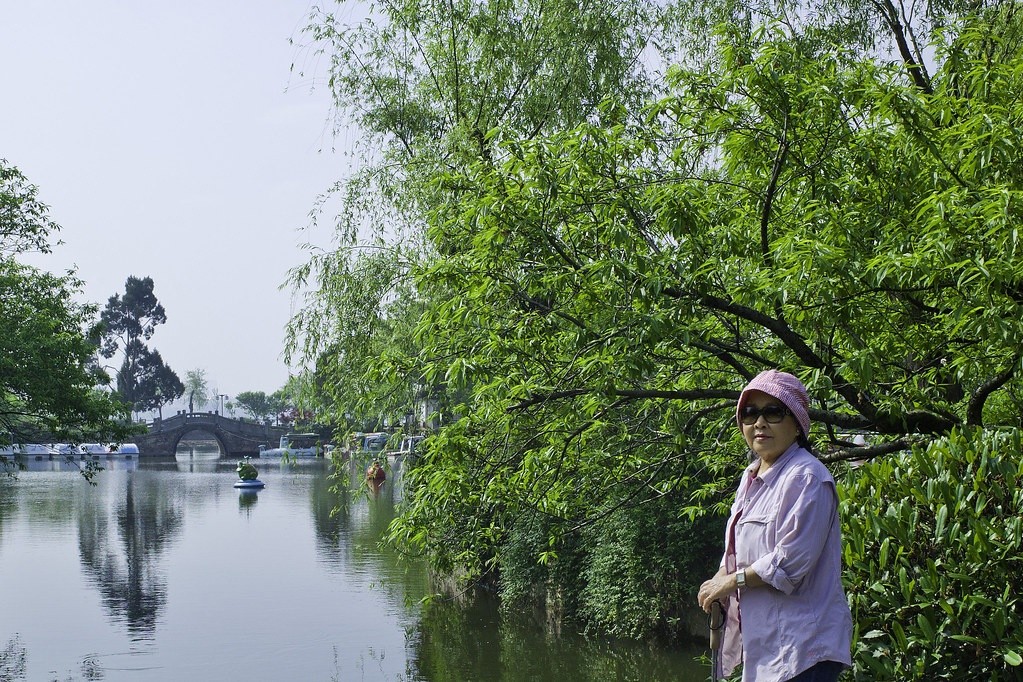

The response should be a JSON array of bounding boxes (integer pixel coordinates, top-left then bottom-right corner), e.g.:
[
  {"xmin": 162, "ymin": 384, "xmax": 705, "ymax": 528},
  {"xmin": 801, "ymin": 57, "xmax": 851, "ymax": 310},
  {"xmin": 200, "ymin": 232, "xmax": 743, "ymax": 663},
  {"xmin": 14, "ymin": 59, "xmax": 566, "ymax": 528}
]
[{"xmin": 741, "ymin": 405, "xmax": 795, "ymax": 426}]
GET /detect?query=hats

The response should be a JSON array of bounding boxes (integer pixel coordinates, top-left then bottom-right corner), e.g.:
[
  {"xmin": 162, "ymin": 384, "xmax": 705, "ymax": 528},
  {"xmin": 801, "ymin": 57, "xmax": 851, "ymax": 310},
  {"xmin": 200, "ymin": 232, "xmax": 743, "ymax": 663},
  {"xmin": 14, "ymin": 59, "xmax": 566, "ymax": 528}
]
[{"xmin": 736, "ymin": 369, "xmax": 810, "ymax": 439}]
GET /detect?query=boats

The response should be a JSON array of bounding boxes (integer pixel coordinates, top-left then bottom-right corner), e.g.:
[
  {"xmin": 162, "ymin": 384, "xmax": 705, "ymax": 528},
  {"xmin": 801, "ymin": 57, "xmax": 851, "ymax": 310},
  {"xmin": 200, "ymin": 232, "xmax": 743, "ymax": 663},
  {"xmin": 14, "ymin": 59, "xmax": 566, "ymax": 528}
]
[
  {"xmin": 106, "ymin": 441, "xmax": 141, "ymax": 457},
  {"xmin": 11, "ymin": 444, "xmax": 49, "ymax": 459},
  {"xmin": 367, "ymin": 467, "xmax": 385, "ymax": 477},
  {"xmin": 257, "ymin": 432, "xmax": 323, "ymax": 460},
  {"xmin": 79, "ymin": 442, "xmax": 108, "ymax": 458},
  {"xmin": 49, "ymin": 443, "xmax": 82, "ymax": 459},
  {"xmin": 324, "ymin": 432, "xmax": 390, "ymax": 460},
  {"xmin": 0, "ymin": 445, "xmax": 15, "ymax": 461},
  {"xmin": 385, "ymin": 436, "xmax": 426, "ymax": 462}
]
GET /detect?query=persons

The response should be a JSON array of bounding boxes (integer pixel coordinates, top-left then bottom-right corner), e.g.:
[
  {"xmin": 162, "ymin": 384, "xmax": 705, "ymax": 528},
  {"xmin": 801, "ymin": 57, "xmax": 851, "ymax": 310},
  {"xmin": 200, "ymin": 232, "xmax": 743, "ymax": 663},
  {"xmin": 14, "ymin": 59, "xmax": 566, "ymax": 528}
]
[
  {"xmin": 368, "ymin": 461, "xmax": 381, "ymax": 474},
  {"xmin": 235, "ymin": 461, "xmax": 258, "ymax": 480},
  {"xmin": 697, "ymin": 370, "xmax": 853, "ymax": 681}
]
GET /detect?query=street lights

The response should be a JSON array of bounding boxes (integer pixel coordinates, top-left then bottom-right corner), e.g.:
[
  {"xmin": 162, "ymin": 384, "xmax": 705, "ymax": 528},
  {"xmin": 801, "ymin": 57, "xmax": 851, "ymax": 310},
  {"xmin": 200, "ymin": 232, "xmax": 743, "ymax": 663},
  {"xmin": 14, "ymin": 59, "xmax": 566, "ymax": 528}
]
[{"xmin": 216, "ymin": 394, "xmax": 229, "ymax": 416}]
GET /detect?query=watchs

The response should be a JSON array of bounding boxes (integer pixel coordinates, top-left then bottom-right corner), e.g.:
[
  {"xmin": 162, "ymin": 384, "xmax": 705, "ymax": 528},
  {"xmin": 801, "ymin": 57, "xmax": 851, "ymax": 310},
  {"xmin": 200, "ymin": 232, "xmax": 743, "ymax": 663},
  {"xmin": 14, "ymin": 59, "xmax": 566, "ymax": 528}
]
[{"xmin": 736, "ymin": 568, "xmax": 749, "ymax": 588}]
